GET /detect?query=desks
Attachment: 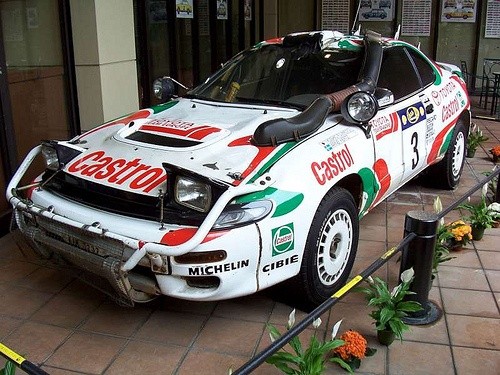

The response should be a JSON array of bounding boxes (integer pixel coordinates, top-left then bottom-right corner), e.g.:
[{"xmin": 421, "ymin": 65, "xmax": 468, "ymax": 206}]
[{"xmin": 479, "ymin": 56, "xmax": 500, "ymax": 116}]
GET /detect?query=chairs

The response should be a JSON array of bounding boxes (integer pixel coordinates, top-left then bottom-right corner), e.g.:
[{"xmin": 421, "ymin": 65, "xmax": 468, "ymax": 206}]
[{"xmin": 460, "ymin": 60, "xmax": 497, "ymax": 110}]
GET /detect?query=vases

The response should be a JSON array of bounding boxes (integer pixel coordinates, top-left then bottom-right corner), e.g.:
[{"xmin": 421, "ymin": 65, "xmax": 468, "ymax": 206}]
[
  {"xmin": 466, "ymin": 147, "xmax": 478, "ymax": 157},
  {"xmin": 470, "ymin": 219, "xmax": 500, "ymax": 239},
  {"xmin": 376, "ymin": 326, "xmax": 396, "ymax": 345}
]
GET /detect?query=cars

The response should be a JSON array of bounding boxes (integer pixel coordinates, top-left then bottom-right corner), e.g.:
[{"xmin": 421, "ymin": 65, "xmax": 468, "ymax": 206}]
[{"xmin": 6, "ymin": 30, "xmax": 472, "ymax": 303}]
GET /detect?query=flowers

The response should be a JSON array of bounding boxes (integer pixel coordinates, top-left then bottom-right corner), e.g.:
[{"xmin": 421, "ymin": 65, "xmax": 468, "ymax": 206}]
[
  {"xmin": 266, "ymin": 160, "xmax": 500, "ymax": 375},
  {"xmin": 465, "ymin": 124, "xmax": 499, "ymax": 158}
]
